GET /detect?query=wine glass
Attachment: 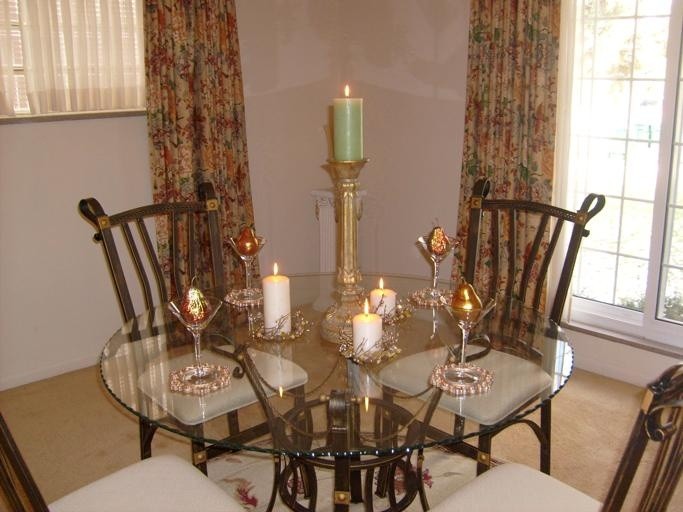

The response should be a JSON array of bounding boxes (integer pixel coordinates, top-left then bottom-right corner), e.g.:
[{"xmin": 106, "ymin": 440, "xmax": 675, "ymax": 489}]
[
  {"xmin": 168, "ymin": 295, "xmax": 223, "ymax": 385},
  {"xmin": 439, "ymin": 294, "xmax": 497, "ymax": 385},
  {"xmin": 424, "ymin": 307, "xmax": 448, "ymax": 347},
  {"xmin": 226, "ymin": 236, "xmax": 267, "ymax": 299},
  {"xmin": 417, "ymin": 235, "xmax": 462, "ymax": 297}
]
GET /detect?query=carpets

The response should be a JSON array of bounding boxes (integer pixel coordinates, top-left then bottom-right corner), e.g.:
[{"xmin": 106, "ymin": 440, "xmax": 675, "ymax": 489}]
[{"xmin": 183, "ymin": 443, "xmax": 501, "ymax": 512}]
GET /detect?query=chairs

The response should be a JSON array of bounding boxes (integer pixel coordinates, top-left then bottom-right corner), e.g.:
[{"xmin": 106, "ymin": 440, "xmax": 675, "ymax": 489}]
[
  {"xmin": 0, "ymin": 408, "xmax": 249, "ymax": 512},
  {"xmin": 78, "ymin": 179, "xmax": 307, "ymax": 477},
  {"xmin": 428, "ymin": 359, "xmax": 683, "ymax": 512},
  {"xmin": 373, "ymin": 175, "xmax": 606, "ymax": 487}
]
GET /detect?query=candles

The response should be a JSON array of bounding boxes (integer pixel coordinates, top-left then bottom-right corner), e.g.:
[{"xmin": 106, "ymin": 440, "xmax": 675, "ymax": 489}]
[
  {"xmin": 353, "ymin": 298, "xmax": 383, "ymax": 359},
  {"xmin": 333, "ymin": 83, "xmax": 363, "ymax": 162},
  {"xmin": 262, "ymin": 263, "xmax": 292, "ymax": 336},
  {"xmin": 369, "ymin": 275, "xmax": 397, "ymax": 315}
]
